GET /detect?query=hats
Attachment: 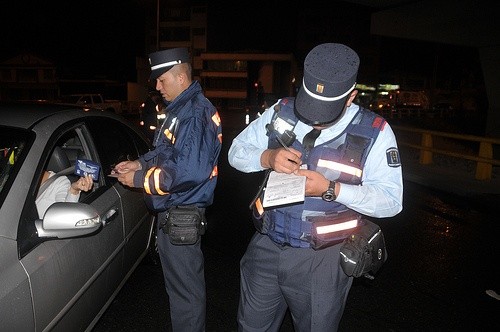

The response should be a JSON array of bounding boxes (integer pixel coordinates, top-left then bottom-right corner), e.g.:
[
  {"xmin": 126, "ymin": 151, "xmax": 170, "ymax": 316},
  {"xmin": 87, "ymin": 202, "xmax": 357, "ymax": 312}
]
[
  {"xmin": 146, "ymin": 46, "xmax": 194, "ymax": 82},
  {"xmin": 295, "ymin": 43, "xmax": 360, "ymax": 126}
]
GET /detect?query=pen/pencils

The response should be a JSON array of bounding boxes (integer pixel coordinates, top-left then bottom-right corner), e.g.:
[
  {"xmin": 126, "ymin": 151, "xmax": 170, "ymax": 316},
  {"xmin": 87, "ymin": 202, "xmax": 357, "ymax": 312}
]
[{"xmin": 274, "ymin": 134, "xmax": 304, "ymax": 168}]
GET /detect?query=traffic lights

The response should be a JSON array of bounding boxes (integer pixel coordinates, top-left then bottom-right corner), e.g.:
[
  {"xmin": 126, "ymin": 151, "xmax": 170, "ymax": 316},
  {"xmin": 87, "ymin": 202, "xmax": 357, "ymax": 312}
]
[{"xmin": 254, "ymin": 82, "xmax": 258, "ymax": 87}]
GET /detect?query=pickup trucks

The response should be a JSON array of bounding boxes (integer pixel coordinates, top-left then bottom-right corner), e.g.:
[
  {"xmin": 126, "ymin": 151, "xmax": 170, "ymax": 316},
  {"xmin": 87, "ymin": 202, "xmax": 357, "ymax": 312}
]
[{"xmin": 55, "ymin": 92, "xmax": 123, "ymax": 115}]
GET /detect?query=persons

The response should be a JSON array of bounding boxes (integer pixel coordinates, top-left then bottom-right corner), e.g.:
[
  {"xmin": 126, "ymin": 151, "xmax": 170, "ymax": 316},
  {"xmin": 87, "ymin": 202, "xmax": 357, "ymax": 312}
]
[
  {"xmin": 227, "ymin": 43, "xmax": 402, "ymax": 332},
  {"xmin": 111, "ymin": 47, "xmax": 222, "ymax": 332},
  {"xmin": 142, "ymin": 89, "xmax": 160, "ymax": 140},
  {"xmin": 0, "ymin": 142, "xmax": 94, "ymax": 220}
]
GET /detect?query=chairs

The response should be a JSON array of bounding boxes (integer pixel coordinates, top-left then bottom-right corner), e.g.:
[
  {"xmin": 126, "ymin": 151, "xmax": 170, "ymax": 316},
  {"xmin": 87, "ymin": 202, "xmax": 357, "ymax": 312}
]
[{"xmin": 47, "ymin": 147, "xmax": 74, "ymax": 183}]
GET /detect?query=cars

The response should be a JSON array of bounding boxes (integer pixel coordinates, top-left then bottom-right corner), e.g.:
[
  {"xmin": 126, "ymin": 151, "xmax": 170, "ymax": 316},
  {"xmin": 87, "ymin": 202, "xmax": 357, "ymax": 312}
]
[{"xmin": 0, "ymin": 101, "xmax": 162, "ymax": 332}]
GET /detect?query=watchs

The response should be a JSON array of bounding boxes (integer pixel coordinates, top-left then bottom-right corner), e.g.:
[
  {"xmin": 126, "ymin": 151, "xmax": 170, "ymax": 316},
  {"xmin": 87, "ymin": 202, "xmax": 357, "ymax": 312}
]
[{"xmin": 322, "ymin": 180, "xmax": 336, "ymax": 202}]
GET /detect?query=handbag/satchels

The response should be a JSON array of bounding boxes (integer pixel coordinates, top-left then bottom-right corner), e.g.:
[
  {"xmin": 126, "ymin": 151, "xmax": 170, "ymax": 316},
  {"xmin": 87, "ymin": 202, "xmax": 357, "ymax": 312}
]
[
  {"xmin": 340, "ymin": 218, "xmax": 389, "ymax": 282},
  {"xmin": 161, "ymin": 205, "xmax": 208, "ymax": 246}
]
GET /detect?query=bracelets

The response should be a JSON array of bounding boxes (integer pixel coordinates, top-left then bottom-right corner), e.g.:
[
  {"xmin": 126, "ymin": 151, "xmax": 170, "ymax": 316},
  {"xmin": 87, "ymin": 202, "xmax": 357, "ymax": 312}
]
[{"xmin": 135, "ymin": 159, "xmax": 142, "ymax": 171}]
